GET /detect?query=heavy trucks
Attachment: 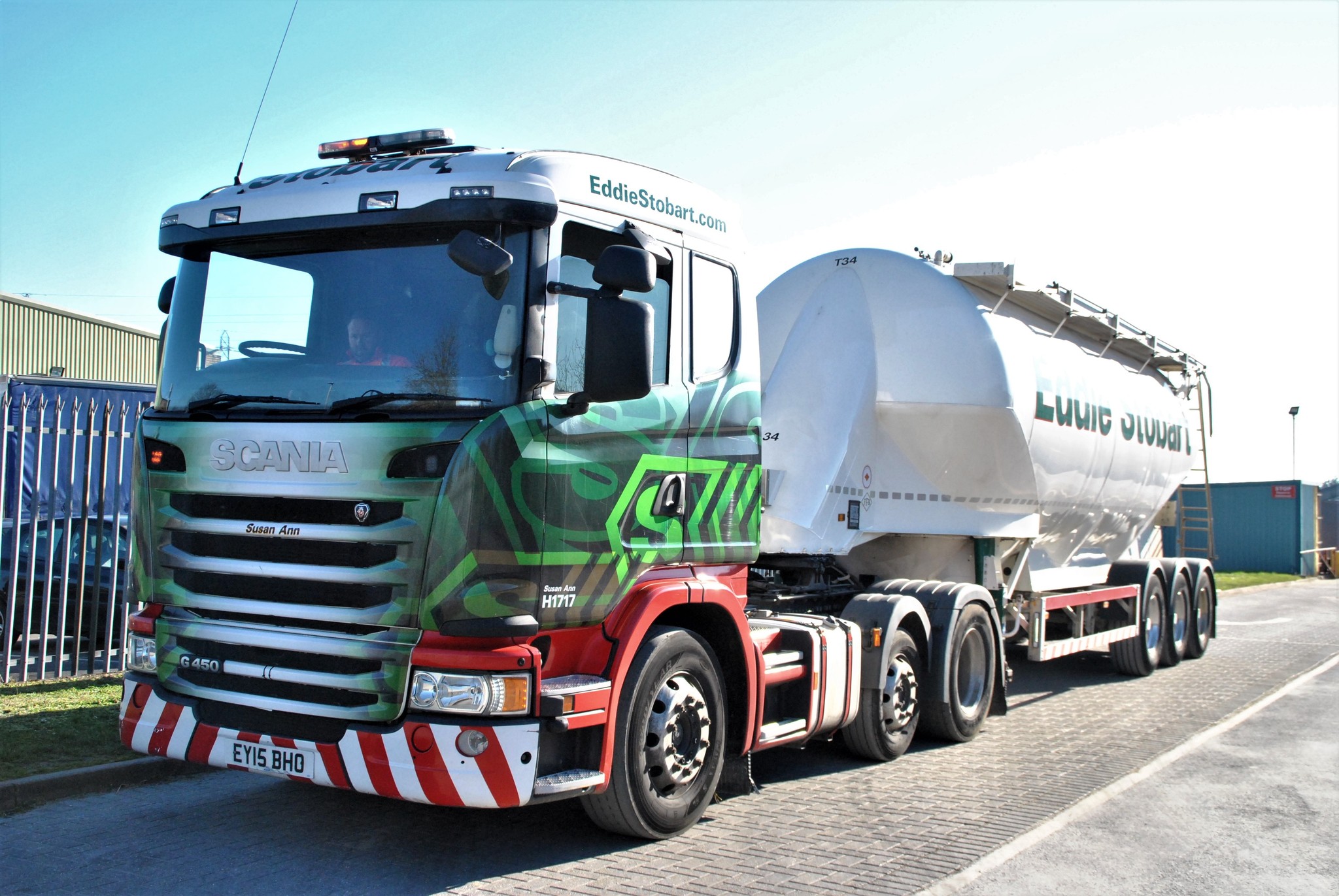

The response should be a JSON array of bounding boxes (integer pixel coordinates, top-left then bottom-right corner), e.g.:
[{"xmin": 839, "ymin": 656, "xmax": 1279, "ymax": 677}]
[{"xmin": 119, "ymin": 133, "xmax": 1218, "ymax": 842}]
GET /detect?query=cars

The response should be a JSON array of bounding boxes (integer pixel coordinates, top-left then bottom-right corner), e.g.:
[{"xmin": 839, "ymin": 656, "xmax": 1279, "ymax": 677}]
[{"xmin": 0, "ymin": 516, "xmax": 128, "ymax": 651}]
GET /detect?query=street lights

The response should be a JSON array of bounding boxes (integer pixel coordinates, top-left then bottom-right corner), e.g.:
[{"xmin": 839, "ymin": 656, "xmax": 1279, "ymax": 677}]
[{"xmin": 1289, "ymin": 406, "xmax": 1298, "ymax": 479}]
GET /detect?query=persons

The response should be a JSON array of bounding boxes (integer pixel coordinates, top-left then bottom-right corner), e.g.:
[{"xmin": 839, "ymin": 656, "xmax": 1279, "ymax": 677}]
[{"xmin": 337, "ymin": 312, "xmax": 412, "ymax": 367}]
